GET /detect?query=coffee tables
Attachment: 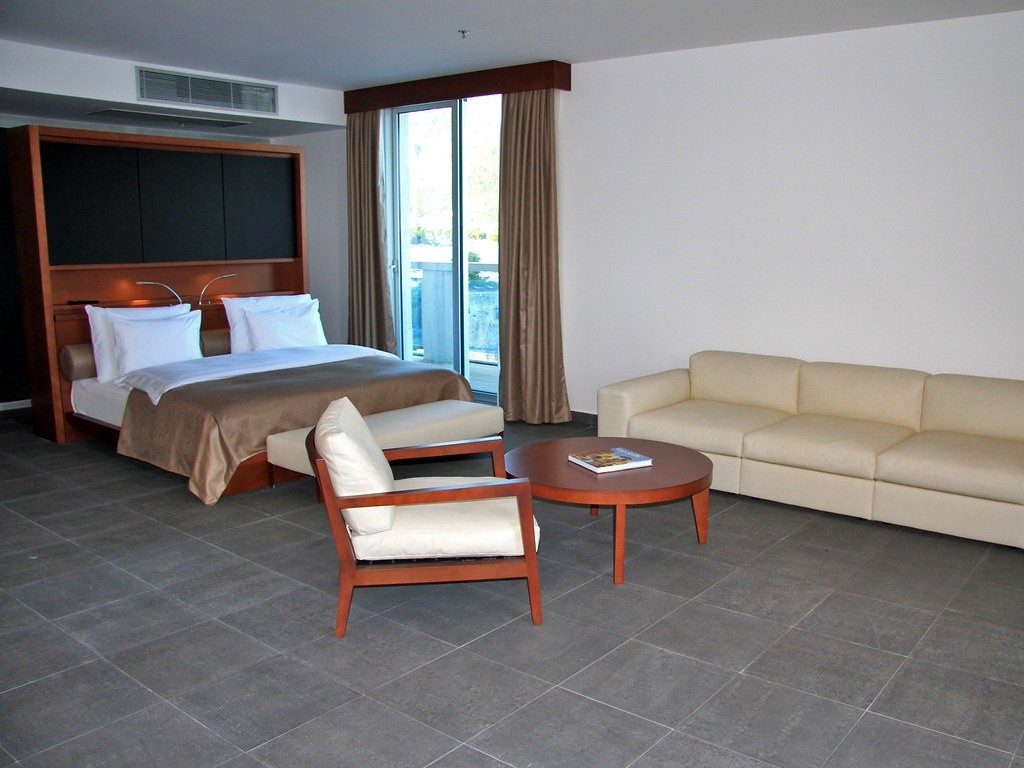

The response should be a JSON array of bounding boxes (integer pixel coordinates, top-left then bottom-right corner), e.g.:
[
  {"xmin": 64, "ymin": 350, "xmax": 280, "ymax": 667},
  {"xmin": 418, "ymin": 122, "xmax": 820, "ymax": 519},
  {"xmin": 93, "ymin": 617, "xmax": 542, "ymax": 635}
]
[{"xmin": 504, "ymin": 437, "xmax": 713, "ymax": 584}]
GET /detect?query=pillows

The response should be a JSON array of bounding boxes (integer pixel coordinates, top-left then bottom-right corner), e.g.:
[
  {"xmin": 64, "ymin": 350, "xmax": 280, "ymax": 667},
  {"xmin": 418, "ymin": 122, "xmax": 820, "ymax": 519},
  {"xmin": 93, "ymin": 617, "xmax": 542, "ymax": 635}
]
[
  {"xmin": 221, "ymin": 294, "xmax": 313, "ymax": 355},
  {"xmin": 107, "ymin": 309, "xmax": 204, "ymax": 379},
  {"xmin": 85, "ymin": 303, "xmax": 198, "ymax": 382},
  {"xmin": 243, "ymin": 298, "xmax": 329, "ymax": 350}
]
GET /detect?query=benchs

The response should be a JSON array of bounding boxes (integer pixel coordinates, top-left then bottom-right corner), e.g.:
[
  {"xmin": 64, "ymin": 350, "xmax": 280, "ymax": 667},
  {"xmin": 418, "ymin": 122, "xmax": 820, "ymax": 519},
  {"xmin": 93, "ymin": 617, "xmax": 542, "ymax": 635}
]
[{"xmin": 266, "ymin": 399, "xmax": 506, "ymax": 504}]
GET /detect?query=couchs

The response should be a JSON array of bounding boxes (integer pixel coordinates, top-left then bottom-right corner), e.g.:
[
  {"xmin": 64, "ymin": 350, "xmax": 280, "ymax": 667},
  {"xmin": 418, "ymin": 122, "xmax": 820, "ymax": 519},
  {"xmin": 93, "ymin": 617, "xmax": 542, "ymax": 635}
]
[{"xmin": 596, "ymin": 349, "xmax": 1023, "ymax": 552}]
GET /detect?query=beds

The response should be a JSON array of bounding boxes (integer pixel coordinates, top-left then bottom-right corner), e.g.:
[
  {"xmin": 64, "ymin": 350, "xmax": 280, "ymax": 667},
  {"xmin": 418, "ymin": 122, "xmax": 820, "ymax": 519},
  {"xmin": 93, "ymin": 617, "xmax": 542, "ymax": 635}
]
[{"xmin": 53, "ymin": 300, "xmax": 476, "ymax": 507}]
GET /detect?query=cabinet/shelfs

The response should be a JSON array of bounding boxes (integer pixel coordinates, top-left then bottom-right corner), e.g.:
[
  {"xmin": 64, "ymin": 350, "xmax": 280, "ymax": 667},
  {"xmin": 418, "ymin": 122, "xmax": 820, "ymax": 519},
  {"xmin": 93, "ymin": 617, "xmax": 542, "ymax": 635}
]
[{"xmin": 4, "ymin": 124, "xmax": 310, "ymax": 442}]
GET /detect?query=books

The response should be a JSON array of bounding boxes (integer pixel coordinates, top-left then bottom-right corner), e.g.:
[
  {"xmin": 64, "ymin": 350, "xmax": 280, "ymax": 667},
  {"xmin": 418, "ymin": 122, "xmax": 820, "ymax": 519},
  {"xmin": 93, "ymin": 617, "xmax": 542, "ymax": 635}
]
[{"xmin": 568, "ymin": 447, "xmax": 653, "ymax": 474}]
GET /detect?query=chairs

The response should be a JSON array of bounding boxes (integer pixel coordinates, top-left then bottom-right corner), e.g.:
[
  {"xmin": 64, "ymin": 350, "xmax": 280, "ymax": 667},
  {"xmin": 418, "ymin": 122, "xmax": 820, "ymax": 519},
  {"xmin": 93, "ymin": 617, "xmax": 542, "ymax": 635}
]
[{"xmin": 305, "ymin": 396, "xmax": 543, "ymax": 636}]
